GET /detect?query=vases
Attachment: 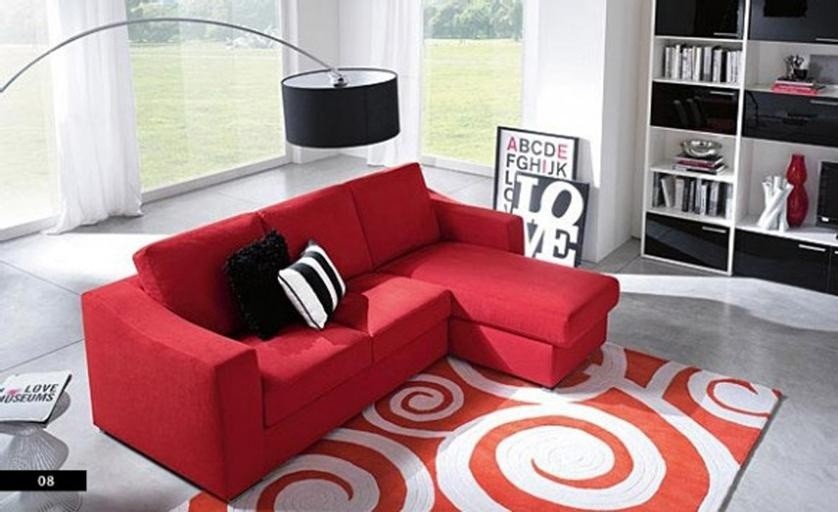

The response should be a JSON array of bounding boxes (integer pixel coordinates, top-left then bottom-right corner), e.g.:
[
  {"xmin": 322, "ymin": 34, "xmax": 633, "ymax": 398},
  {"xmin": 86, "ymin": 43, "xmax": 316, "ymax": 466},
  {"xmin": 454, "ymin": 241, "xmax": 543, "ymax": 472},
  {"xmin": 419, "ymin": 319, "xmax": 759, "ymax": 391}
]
[{"xmin": 782, "ymin": 153, "xmax": 808, "ymax": 228}]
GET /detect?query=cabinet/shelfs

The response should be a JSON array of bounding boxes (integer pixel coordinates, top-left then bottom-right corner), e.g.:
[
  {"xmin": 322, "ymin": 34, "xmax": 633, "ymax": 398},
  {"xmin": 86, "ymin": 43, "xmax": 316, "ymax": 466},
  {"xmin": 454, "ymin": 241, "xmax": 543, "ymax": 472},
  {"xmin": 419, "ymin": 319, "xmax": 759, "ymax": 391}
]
[{"xmin": 641, "ymin": 0, "xmax": 837, "ymax": 295}]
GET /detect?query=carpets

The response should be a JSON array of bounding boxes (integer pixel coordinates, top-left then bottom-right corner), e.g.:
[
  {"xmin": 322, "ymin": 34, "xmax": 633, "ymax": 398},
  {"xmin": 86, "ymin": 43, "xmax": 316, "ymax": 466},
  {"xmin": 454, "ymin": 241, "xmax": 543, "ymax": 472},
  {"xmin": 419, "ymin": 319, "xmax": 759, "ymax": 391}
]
[{"xmin": 168, "ymin": 340, "xmax": 782, "ymax": 512}]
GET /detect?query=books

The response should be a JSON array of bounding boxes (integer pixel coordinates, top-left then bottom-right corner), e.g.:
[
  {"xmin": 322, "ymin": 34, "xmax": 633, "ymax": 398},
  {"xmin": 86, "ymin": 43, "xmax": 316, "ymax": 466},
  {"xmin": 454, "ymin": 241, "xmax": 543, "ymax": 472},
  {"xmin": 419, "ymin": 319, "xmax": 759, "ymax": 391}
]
[
  {"xmin": 0, "ymin": 368, "xmax": 74, "ymax": 427},
  {"xmin": 771, "ymin": 84, "xmax": 826, "ymax": 96},
  {"xmin": 658, "ymin": 41, "xmax": 742, "ymax": 84},
  {"xmin": 774, "ymin": 76, "xmax": 818, "ymax": 87},
  {"xmin": 649, "ymin": 154, "xmax": 734, "ymax": 219}
]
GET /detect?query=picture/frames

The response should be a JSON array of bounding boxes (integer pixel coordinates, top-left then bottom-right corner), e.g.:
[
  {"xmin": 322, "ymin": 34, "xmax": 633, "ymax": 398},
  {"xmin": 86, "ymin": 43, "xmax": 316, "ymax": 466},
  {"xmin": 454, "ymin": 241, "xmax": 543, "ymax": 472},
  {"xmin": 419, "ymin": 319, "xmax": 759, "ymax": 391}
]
[{"xmin": 493, "ymin": 125, "xmax": 581, "ymax": 215}]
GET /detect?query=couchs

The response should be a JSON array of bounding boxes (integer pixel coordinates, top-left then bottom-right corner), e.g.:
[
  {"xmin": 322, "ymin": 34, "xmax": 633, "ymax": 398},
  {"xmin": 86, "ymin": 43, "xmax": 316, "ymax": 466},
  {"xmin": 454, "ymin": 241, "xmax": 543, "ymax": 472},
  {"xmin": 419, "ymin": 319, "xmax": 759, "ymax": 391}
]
[{"xmin": 81, "ymin": 159, "xmax": 622, "ymax": 505}]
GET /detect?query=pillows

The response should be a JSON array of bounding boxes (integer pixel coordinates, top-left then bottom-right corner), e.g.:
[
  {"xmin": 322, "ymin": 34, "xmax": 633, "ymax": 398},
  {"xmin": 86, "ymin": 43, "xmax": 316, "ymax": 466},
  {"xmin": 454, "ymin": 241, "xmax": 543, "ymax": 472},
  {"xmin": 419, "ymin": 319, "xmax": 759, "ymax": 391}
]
[
  {"xmin": 226, "ymin": 230, "xmax": 298, "ymax": 340},
  {"xmin": 276, "ymin": 239, "xmax": 347, "ymax": 332}
]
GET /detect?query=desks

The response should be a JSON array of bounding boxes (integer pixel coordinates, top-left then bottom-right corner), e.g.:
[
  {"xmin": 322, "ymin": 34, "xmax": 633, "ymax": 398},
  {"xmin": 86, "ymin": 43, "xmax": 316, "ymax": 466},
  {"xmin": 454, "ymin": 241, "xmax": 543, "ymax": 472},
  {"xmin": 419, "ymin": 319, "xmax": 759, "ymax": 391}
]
[{"xmin": 0, "ymin": 386, "xmax": 83, "ymax": 511}]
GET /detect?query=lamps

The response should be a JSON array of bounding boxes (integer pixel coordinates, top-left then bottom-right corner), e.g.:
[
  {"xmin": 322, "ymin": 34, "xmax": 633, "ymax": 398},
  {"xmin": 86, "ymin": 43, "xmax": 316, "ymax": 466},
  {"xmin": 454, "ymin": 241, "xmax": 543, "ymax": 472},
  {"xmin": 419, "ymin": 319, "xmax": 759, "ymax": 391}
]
[{"xmin": 0, "ymin": 17, "xmax": 400, "ymax": 148}]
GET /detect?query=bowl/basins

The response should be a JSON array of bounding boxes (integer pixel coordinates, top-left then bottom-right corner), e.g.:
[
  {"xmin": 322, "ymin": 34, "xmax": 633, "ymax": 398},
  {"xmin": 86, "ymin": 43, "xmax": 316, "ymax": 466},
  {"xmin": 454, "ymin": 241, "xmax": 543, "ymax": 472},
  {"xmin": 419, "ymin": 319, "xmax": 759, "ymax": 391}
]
[{"xmin": 680, "ymin": 139, "xmax": 720, "ymax": 157}]
